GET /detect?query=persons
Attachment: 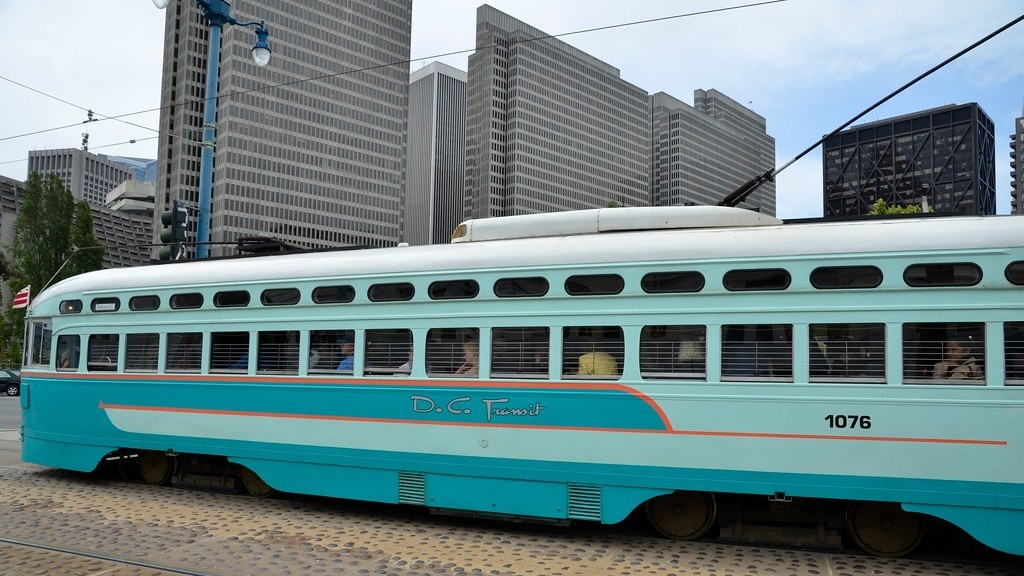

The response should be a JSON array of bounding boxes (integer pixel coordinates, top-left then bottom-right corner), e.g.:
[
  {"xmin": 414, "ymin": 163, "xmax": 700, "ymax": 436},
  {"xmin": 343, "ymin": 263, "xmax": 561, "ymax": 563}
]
[
  {"xmin": 578, "ymin": 336, "xmax": 618, "ymax": 375},
  {"xmin": 57, "ymin": 339, "xmax": 79, "ymax": 368},
  {"xmin": 336, "ymin": 343, "xmax": 372, "ymax": 370},
  {"xmin": 230, "ymin": 344, "xmax": 283, "ymax": 371},
  {"xmin": 932, "ymin": 336, "xmax": 982, "ymax": 379},
  {"xmin": 455, "ymin": 340, "xmax": 479, "ymax": 374},
  {"xmin": 535, "ymin": 348, "xmax": 549, "ymax": 372},
  {"xmin": 393, "ymin": 351, "xmax": 413, "ymax": 375}
]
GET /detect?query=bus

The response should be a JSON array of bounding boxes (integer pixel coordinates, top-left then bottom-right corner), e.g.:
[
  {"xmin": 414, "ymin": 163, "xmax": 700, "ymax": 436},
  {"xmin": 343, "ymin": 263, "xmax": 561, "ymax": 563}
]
[{"xmin": 20, "ymin": 10, "xmax": 1024, "ymax": 560}]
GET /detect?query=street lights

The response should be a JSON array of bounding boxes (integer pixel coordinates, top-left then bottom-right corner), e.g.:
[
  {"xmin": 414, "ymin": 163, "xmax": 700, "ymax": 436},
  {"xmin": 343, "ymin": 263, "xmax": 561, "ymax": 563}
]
[{"xmin": 152, "ymin": 1, "xmax": 272, "ymax": 260}]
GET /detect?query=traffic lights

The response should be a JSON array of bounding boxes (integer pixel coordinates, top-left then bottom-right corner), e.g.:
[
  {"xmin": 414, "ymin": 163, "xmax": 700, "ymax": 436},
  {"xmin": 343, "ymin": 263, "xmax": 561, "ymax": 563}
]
[{"xmin": 159, "ymin": 209, "xmax": 187, "ymax": 262}]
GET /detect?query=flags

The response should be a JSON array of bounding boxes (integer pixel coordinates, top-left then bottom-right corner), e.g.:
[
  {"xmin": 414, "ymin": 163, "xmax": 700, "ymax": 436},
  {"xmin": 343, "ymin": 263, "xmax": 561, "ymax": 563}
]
[{"xmin": 12, "ymin": 287, "xmax": 28, "ymax": 309}]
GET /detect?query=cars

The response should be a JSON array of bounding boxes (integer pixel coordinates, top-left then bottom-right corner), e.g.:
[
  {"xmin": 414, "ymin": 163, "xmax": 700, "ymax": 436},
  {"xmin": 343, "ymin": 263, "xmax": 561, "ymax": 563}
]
[{"xmin": 0, "ymin": 369, "xmax": 20, "ymax": 397}]
